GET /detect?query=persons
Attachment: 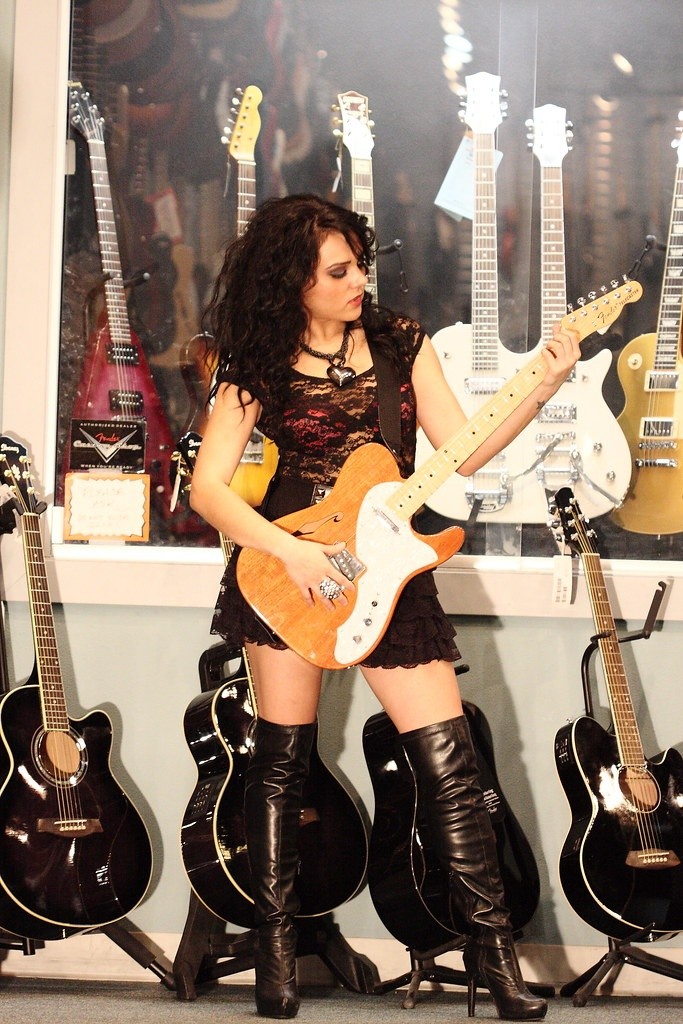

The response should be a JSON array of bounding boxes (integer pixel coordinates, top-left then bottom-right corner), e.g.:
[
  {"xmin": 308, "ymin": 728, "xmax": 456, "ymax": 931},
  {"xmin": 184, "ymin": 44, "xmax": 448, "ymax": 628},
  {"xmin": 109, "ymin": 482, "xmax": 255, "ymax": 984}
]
[{"xmin": 188, "ymin": 194, "xmax": 581, "ymax": 1020}]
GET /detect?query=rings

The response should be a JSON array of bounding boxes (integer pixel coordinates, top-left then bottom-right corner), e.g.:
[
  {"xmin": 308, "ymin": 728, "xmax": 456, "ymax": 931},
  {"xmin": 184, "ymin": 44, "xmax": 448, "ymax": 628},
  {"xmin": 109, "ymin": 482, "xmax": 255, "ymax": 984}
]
[{"xmin": 320, "ymin": 579, "xmax": 341, "ymax": 599}]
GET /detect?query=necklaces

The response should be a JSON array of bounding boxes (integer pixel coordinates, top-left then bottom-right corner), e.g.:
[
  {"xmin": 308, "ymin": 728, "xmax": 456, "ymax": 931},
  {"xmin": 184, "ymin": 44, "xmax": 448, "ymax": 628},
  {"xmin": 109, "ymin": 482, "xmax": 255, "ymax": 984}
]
[{"xmin": 298, "ymin": 323, "xmax": 356, "ymax": 386}]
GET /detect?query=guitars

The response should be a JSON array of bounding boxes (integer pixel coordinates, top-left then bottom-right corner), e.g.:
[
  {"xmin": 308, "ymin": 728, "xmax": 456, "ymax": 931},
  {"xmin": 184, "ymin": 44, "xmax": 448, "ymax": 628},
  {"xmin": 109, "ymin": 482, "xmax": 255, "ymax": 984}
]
[
  {"xmin": 169, "ymin": 80, "xmax": 279, "ymax": 545},
  {"xmin": 546, "ymin": 488, "xmax": 683, "ymax": 941},
  {"xmin": 363, "ymin": 665, "xmax": 540, "ymax": 952},
  {"xmin": 172, "ymin": 431, "xmax": 372, "ymax": 928},
  {"xmin": 169, "ymin": 82, "xmax": 279, "ymax": 508},
  {"xmin": 0, "ymin": 436, "xmax": 155, "ymax": 942},
  {"xmin": 525, "ymin": 103, "xmax": 632, "ymax": 526},
  {"xmin": 333, "ymin": 91, "xmax": 382, "ymax": 309},
  {"xmin": 65, "ymin": 78, "xmax": 173, "ymax": 543},
  {"xmin": 415, "ymin": 68, "xmax": 564, "ymax": 526},
  {"xmin": 607, "ymin": 111, "xmax": 683, "ymax": 535},
  {"xmin": 237, "ymin": 272, "xmax": 642, "ymax": 672}
]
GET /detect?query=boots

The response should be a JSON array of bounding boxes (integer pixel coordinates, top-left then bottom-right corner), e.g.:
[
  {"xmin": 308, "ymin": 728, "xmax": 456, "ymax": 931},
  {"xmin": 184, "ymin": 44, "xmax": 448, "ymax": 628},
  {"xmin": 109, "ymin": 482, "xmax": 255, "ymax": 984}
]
[
  {"xmin": 398, "ymin": 714, "xmax": 547, "ymax": 1022},
  {"xmin": 245, "ymin": 715, "xmax": 318, "ymax": 1019}
]
